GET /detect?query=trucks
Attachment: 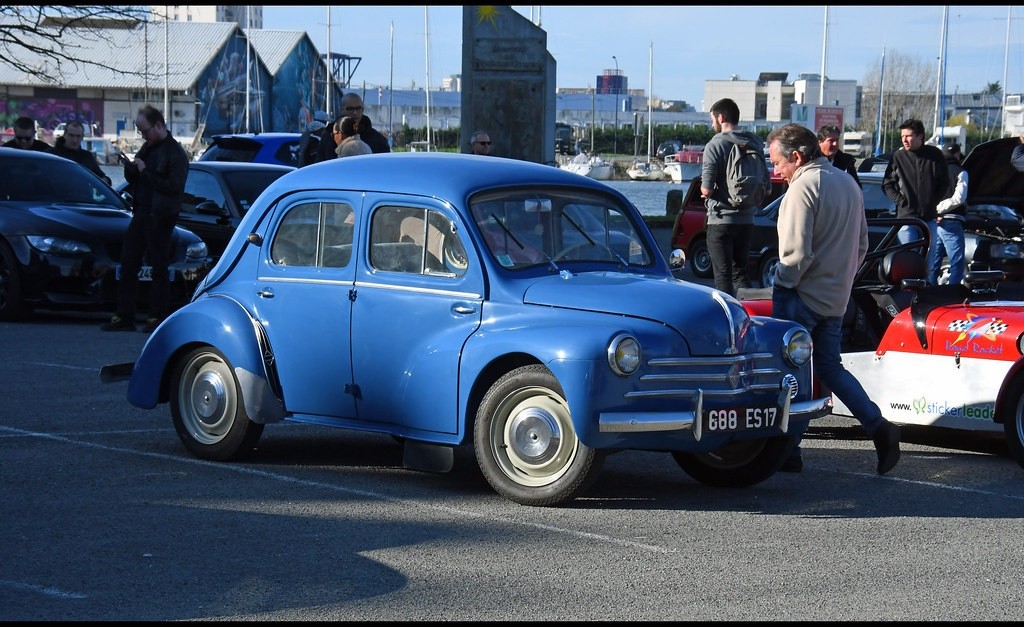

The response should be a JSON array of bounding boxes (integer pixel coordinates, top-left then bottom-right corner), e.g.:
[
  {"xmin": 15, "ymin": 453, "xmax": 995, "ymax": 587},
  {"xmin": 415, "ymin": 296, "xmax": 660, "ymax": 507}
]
[
  {"xmin": 843, "ymin": 131, "xmax": 873, "ymax": 158},
  {"xmin": 934, "ymin": 125, "xmax": 967, "ymax": 159},
  {"xmin": 555, "ymin": 122, "xmax": 575, "ymax": 155}
]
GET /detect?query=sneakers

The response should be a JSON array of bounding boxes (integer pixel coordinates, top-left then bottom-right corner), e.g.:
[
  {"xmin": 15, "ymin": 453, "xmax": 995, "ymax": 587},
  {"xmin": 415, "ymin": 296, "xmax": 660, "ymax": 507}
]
[
  {"xmin": 100, "ymin": 316, "xmax": 135, "ymax": 331},
  {"xmin": 143, "ymin": 318, "xmax": 160, "ymax": 333}
]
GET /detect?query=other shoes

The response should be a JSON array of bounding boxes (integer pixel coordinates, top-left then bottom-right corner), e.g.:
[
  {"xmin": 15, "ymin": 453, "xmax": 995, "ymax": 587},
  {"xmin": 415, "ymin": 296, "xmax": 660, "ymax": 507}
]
[
  {"xmin": 779, "ymin": 448, "xmax": 802, "ymax": 472},
  {"xmin": 870, "ymin": 420, "xmax": 900, "ymax": 474}
]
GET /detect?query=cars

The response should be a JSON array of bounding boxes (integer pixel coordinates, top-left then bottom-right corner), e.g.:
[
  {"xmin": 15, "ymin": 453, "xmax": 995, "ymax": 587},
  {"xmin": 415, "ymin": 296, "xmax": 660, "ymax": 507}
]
[
  {"xmin": 110, "ymin": 159, "xmax": 330, "ymax": 269},
  {"xmin": 0, "ymin": 145, "xmax": 214, "ymax": 321},
  {"xmin": 97, "ymin": 150, "xmax": 834, "ymax": 509},
  {"xmin": 194, "ymin": 130, "xmax": 321, "ymax": 168},
  {"xmin": 656, "ymin": 135, "xmax": 1024, "ymax": 468}
]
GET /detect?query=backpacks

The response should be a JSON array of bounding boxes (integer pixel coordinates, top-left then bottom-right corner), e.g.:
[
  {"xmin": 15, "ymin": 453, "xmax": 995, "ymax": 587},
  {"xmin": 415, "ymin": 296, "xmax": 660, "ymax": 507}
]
[{"xmin": 719, "ymin": 143, "xmax": 767, "ymax": 210}]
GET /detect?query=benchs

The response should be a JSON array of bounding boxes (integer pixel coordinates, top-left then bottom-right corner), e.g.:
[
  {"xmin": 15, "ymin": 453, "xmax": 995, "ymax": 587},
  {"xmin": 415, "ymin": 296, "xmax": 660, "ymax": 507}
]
[{"xmin": 322, "ymin": 243, "xmax": 423, "ymax": 274}]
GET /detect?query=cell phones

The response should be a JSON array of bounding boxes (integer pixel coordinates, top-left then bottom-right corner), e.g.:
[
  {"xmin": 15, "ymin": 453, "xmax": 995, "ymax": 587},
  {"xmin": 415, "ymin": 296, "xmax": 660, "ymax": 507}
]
[{"xmin": 116, "ymin": 153, "xmax": 129, "ymax": 161}]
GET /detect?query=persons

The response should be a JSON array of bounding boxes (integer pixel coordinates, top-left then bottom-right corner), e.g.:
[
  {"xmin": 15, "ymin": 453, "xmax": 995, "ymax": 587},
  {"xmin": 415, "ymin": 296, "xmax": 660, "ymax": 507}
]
[
  {"xmin": 299, "ymin": 111, "xmax": 332, "ymax": 168},
  {"xmin": 880, "ymin": 119, "xmax": 947, "ymax": 281},
  {"xmin": 468, "ymin": 130, "xmax": 491, "ymax": 154},
  {"xmin": 699, "ymin": 98, "xmax": 773, "ymax": 297},
  {"xmin": 333, "ymin": 116, "xmax": 372, "ymax": 157},
  {"xmin": 0, "ymin": 117, "xmax": 52, "ymax": 152},
  {"xmin": 48, "ymin": 120, "xmax": 112, "ymax": 187},
  {"xmin": 765, "ymin": 123, "xmax": 900, "ymax": 475},
  {"xmin": 932, "ymin": 140, "xmax": 968, "ymax": 285},
  {"xmin": 315, "ymin": 92, "xmax": 390, "ymax": 165},
  {"xmin": 101, "ymin": 105, "xmax": 189, "ymax": 332},
  {"xmin": 816, "ymin": 125, "xmax": 862, "ymax": 188}
]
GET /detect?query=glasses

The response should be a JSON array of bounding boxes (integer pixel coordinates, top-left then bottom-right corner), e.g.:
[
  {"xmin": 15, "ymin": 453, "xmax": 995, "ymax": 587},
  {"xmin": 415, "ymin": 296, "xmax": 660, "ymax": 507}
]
[
  {"xmin": 15, "ymin": 132, "xmax": 33, "ymax": 141},
  {"xmin": 344, "ymin": 106, "xmax": 364, "ymax": 113},
  {"xmin": 137, "ymin": 125, "xmax": 155, "ymax": 135},
  {"xmin": 474, "ymin": 141, "xmax": 491, "ymax": 145}
]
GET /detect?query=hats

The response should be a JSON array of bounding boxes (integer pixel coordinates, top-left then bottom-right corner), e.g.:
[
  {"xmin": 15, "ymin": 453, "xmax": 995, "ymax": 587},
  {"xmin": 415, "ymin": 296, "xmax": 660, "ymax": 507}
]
[
  {"xmin": 308, "ymin": 121, "xmax": 327, "ymax": 132},
  {"xmin": 314, "ymin": 111, "xmax": 331, "ymax": 121}
]
